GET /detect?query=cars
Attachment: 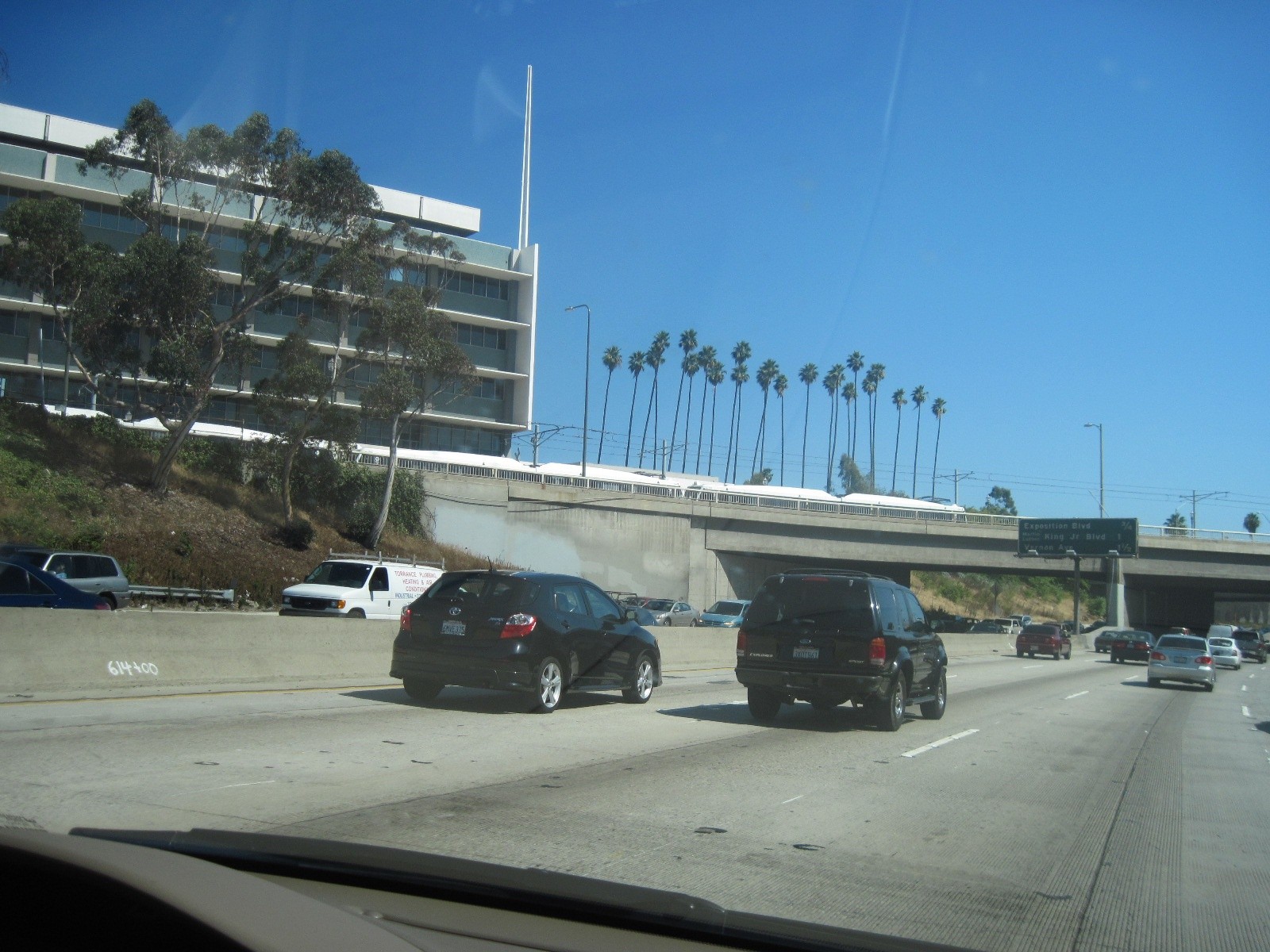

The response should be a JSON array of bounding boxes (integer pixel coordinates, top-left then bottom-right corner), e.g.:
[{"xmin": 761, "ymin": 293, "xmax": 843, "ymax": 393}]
[
  {"xmin": 388, "ymin": 568, "xmax": 664, "ymax": 713},
  {"xmin": 0, "ymin": 548, "xmax": 132, "ymax": 610},
  {"xmin": 1207, "ymin": 637, "xmax": 1242, "ymax": 670},
  {"xmin": 930, "ymin": 617, "xmax": 1022, "ymax": 635},
  {"xmin": 634, "ymin": 599, "xmax": 701, "ymax": 627},
  {"xmin": 1111, "ymin": 630, "xmax": 1157, "ymax": 664},
  {"xmin": 1063, "ymin": 621, "xmax": 1085, "ymax": 633},
  {"xmin": 0, "ymin": 556, "xmax": 112, "ymax": 611},
  {"xmin": 1042, "ymin": 621, "xmax": 1074, "ymax": 634},
  {"xmin": 697, "ymin": 599, "xmax": 753, "ymax": 629},
  {"xmin": 611, "ymin": 596, "xmax": 657, "ymax": 623},
  {"xmin": 1083, "ymin": 621, "xmax": 1106, "ymax": 634},
  {"xmin": 1093, "ymin": 630, "xmax": 1118, "ymax": 654},
  {"xmin": 1147, "ymin": 634, "xmax": 1217, "ymax": 692},
  {"xmin": 1016, "ymin": 624, "xmax": 1072, "ymax": 661}
]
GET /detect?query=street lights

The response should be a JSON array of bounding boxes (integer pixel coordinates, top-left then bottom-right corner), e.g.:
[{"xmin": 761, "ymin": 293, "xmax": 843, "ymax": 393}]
[
  {"xmin": 564, "ymin": 303, "xmax": 590, "ymax": 480},
  {"xmin": 1175, "ymin": 500, "xmax": 1193, "ymax": 536},
  {"xmin": 1083, "ymin": 423, "xmax": 1104, "ymax": 520}
]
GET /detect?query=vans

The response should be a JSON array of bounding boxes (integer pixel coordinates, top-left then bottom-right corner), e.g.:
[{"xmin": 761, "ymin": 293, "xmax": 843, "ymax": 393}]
[{"xmin": 1207, "ymin": 624, "xmax": 1239, "ymax": 640}]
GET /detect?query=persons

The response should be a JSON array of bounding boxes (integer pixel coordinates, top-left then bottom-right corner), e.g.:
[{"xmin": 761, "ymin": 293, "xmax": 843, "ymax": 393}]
[{"xmin": 52, "ymin": 562, "xmax": 68, "ymax": 579}]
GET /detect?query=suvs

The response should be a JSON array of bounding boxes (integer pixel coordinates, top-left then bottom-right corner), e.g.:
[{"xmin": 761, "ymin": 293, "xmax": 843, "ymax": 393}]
[
  {"xmin": 275, "ymin": 548, "xmax": 448, "ymax": 618},
  {"xmin": 1231, "ymin": 630, "xmax": 1269, "ymax": 663},
  {"xmin": 1170, "ymin": 627, "xmax": 1192, "ymax": 636},
  {"xmin": 1009, "ymin": 615, "xmax": 1032, "ymax": 628},
  {"xmin": 734, "ymin": 573, "xmax": 949, "ymax": 734}
]
